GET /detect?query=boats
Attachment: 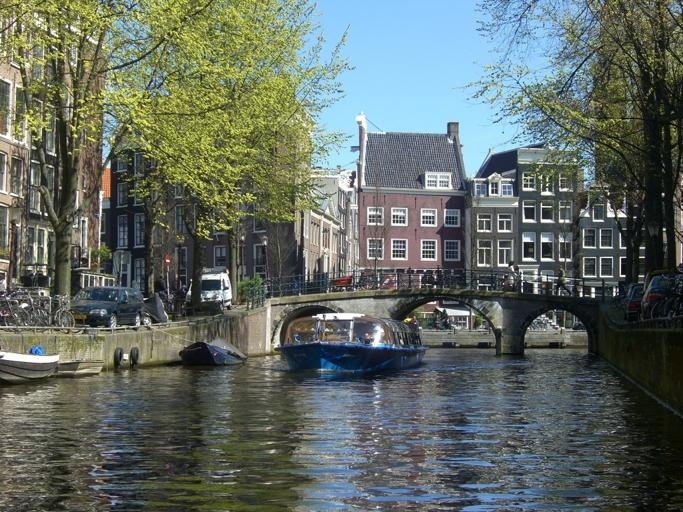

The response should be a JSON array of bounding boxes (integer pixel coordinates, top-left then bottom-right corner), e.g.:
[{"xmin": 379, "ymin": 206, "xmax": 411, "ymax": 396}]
[
  {"xmin": 0, "ymin": 348, "xmax": 61, "ymax": 385},
  {"xmin": 58, "ymin": 359, "xmax": 106, "ymax": 378},
  {"xmin": 273, "ymin": 312, "xmax": 430, "ymax": 377},
  {"xmin": 178, "ymin": 336, "xmax": 248, "ymax": 366}
]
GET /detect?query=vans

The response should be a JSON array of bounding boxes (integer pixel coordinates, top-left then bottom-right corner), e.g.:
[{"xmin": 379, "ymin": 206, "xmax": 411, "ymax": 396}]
[{"xmin": 185, "ymin": 272, "xmax": 232, "ymax": 312}]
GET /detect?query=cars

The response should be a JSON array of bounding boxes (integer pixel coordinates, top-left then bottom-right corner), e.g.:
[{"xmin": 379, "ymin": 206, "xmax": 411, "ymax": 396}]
[
  {"xmin": 641, "ymin": 275, "xmax": 670, "ymax": 314},
  {"xmin": 625, "ymin": 283, "xmax": 643, "ymax": 318},
  {"xmin": 71, "ymin": 287, "xmax": 145, "ymax": 329}
]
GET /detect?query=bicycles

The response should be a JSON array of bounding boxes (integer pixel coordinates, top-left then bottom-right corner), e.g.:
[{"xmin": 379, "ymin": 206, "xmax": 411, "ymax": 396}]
[
  {"xmin": 651, "ymin": 275, "xmax": 683, "ymax": 320},
  {"xmin": 1, "ymin": 291, "xmax": 75, "ymax": 334}
]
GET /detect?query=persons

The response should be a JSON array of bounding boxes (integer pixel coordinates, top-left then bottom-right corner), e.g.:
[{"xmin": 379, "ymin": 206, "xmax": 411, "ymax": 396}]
[
  {"xmin": 555, "ymin": 265, "xmax": 571, "ymax": 296},
  {"xmin": 155, "ymin": 273, "xmax": 166, "ymax": 291},
  {"xmin": 507, "ymin": 261, "xmax": 517, "ymax": 291},
  {"xmin": 410, "ymin": 316, "xmax": 423, "ymax": 346}
]
